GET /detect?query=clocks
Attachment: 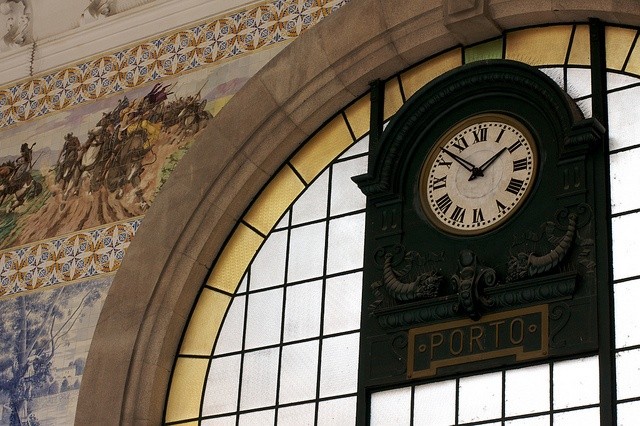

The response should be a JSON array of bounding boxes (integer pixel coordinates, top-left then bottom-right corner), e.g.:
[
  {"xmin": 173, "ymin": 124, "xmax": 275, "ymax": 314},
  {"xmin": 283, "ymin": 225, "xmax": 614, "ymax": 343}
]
[{"xmin": 418, "ymin": 111, "xmax": 539, "ymax": 236}]
